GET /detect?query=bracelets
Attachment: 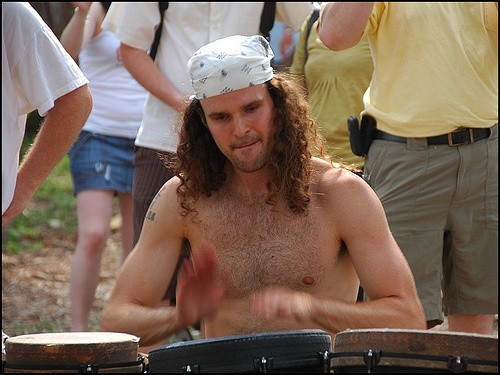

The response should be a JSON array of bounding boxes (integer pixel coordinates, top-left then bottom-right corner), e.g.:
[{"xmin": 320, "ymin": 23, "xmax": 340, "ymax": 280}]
[{"xmin": 74, "ymin": 7, "xmax": 91, "ymax": 24}]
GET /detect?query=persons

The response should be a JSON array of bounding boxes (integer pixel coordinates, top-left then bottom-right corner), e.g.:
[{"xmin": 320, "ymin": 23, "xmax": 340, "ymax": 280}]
[
  {"xmin": 0, "ymin": 2, "xmax": 499, "ymax": 337},
  {"xmin": 100, "ymin": 34, "xmax": 428, "ymax": 353}
]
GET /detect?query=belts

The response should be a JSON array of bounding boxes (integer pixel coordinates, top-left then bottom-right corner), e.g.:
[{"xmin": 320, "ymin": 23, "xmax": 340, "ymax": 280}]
[{"xmin": 373, "ymin": 128, "xmax": 491, "ymax": 147}]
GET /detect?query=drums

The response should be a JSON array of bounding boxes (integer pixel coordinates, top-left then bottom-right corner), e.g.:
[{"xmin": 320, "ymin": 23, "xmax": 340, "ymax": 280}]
[
  {"xmin": 2, "ymin": 331, "xmax": 147, "ymax": 375},
  {"xmin": 144, "ymin": 329, "xmax": 331, "ymax": 375},
  {"xmin": 330, "ymin": 329, "xmax": 499, "ymax": 375}
]
